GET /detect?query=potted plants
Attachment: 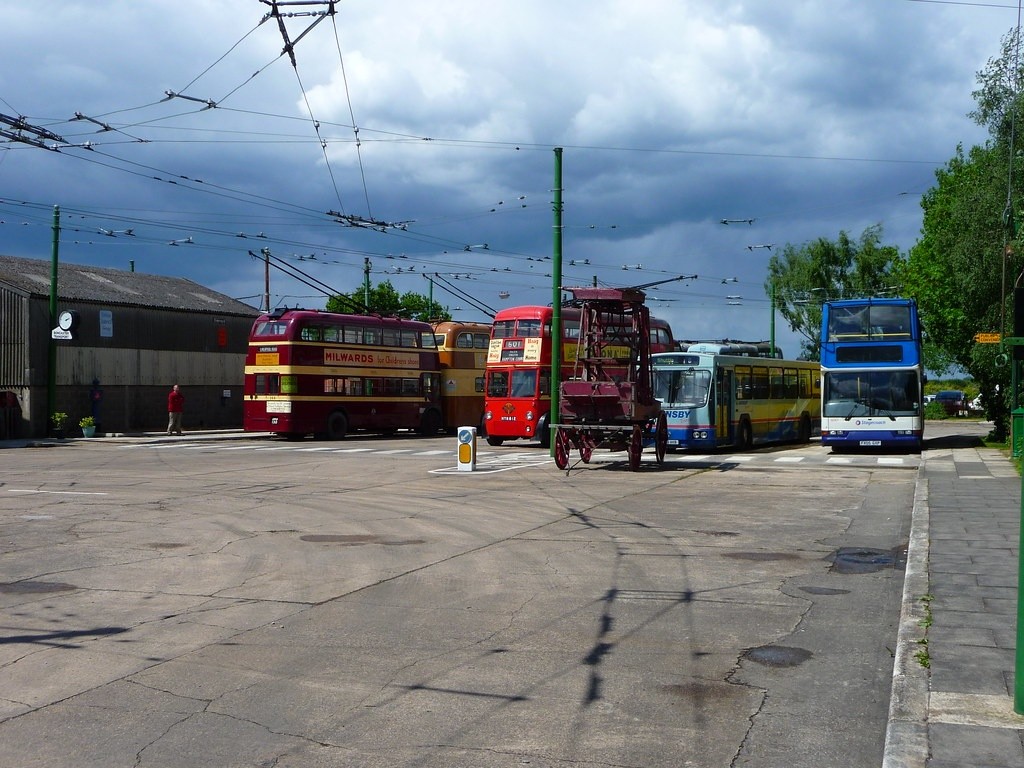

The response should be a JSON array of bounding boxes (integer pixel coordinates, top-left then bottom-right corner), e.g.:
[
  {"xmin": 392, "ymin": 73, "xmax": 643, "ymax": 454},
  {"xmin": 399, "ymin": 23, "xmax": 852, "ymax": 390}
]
[
  {"xmin": 51, "ymin": 413, "xmax": 68, "ymax": 439},
  {"xmin": 79, "ymin": 416, "xmax": 95, "ymax": 437}
]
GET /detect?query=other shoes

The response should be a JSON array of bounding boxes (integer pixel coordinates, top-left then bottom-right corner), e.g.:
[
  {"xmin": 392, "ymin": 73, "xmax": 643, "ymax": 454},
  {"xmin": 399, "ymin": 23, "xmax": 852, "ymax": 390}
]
[
  {"xmin": 168, "ymin": 432, "xmax": 171, "ymax": 435},
  {"xmin": 176, "ymin": 433, "xmax": 185, "ymax": 436}
]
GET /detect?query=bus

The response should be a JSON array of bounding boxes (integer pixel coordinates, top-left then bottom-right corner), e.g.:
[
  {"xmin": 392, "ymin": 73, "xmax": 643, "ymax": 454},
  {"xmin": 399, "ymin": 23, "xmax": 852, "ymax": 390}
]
[
  {"xmin": 243, "ymin": 308, "xmax": 445, "ymax": 441},
  {"xmin": 413, "ymin": 320, "xmax": 552, "ymax": 437},
  {"xmin": 481, "ymin": 305, "xmax": 675, "ymax": 449},
  {"xmin": 636, "ymin": 343, "xmax": 822, "ymax": 452},
  {"xmin": 674, "ymin": 340, "xmax": 783, "ymax": 359},
  {"xmin": 820, "ymin": 298, "xmax": 927, "ymax": 454}
]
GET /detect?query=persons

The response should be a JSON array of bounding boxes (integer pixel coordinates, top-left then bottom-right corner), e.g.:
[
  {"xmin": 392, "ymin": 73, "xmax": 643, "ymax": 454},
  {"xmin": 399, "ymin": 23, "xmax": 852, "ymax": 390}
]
[
  {"xmin": 167, "ymin": 384, "xmax": 185, "ymax": 436},
  {"xmin": 88, "ymin": 378, "xmax": 103, "ymax": 429}
]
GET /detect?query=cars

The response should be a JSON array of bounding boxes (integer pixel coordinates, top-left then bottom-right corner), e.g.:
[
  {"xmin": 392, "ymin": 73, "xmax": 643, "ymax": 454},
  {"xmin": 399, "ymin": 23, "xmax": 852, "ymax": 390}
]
[
  {"xmin": 934, "ymin": 391, "xmax": 967, "ymax": 414},
  {"xmin": 924, "ymin": 395, "xmax": 937, "ymax": 407}
]
